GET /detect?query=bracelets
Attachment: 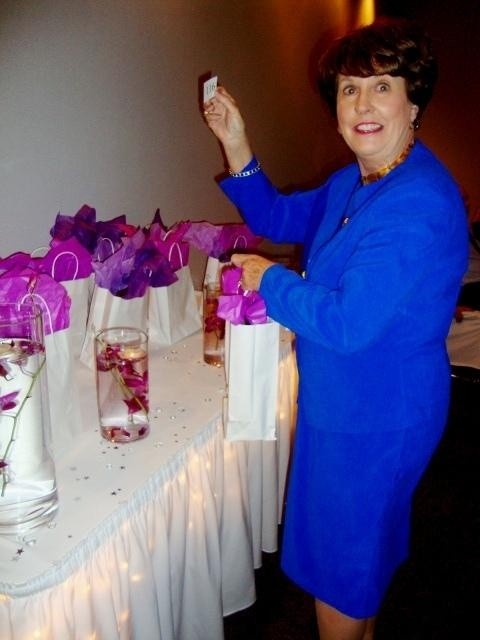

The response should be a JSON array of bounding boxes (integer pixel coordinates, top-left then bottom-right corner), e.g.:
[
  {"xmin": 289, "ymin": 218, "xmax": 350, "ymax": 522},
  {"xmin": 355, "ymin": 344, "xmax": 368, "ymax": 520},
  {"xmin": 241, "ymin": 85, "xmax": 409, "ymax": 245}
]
[{"xmin": 227, "ymin": 161, "xmax": 262, "ymax": 177}]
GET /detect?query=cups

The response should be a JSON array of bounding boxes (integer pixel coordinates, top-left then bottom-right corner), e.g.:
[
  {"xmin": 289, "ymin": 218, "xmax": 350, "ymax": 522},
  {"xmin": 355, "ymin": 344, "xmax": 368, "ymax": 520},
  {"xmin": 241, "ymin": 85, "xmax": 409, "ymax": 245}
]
[
  {"xmin": 202, "ymin": 281, "xmax": 226, "ymax": 368},
  {"xmin": 0, "ymin": 301, "xmax": 61, "ymax": 538},
  {"xmin": 93, "ymin": 327, "xmax": 151, "ymax": 445}
]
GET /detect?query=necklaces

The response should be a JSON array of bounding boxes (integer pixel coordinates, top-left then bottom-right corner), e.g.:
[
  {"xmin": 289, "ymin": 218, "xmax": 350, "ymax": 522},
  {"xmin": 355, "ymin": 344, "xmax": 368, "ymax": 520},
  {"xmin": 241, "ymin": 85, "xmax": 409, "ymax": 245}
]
[{"xmin": 359, "ymin": 132, "xmax": 416, "ymax": 184}]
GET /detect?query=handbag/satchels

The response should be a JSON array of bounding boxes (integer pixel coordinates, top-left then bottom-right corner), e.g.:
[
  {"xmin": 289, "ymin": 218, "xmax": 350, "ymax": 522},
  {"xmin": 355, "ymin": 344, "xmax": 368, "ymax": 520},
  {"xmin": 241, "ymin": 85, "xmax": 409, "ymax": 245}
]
[
  {"xmin": 223, "ymin": 318, "xmax": 282, "ymax": 444},
  {"xmin": 43, "ymin": 262, "xmax": 201, "ymax": 456},
  {"xmin": 205, "ymin": 236, "xmax": 253, "ymax": 286}
]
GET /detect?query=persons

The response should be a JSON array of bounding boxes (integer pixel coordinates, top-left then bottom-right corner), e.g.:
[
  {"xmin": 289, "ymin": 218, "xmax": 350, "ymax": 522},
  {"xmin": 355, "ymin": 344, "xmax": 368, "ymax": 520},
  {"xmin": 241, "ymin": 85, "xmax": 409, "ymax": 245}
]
[{"xmin": 204, "ymin": 24, "xmax": 470, "ymax": 639}]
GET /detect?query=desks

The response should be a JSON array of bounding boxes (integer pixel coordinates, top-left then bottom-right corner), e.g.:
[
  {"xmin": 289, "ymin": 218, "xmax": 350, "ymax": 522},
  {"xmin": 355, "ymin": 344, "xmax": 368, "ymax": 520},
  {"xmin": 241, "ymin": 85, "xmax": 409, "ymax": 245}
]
[{"xmin": 0, "ymin": 320, "xmax": 299, "ymax": 640}]
